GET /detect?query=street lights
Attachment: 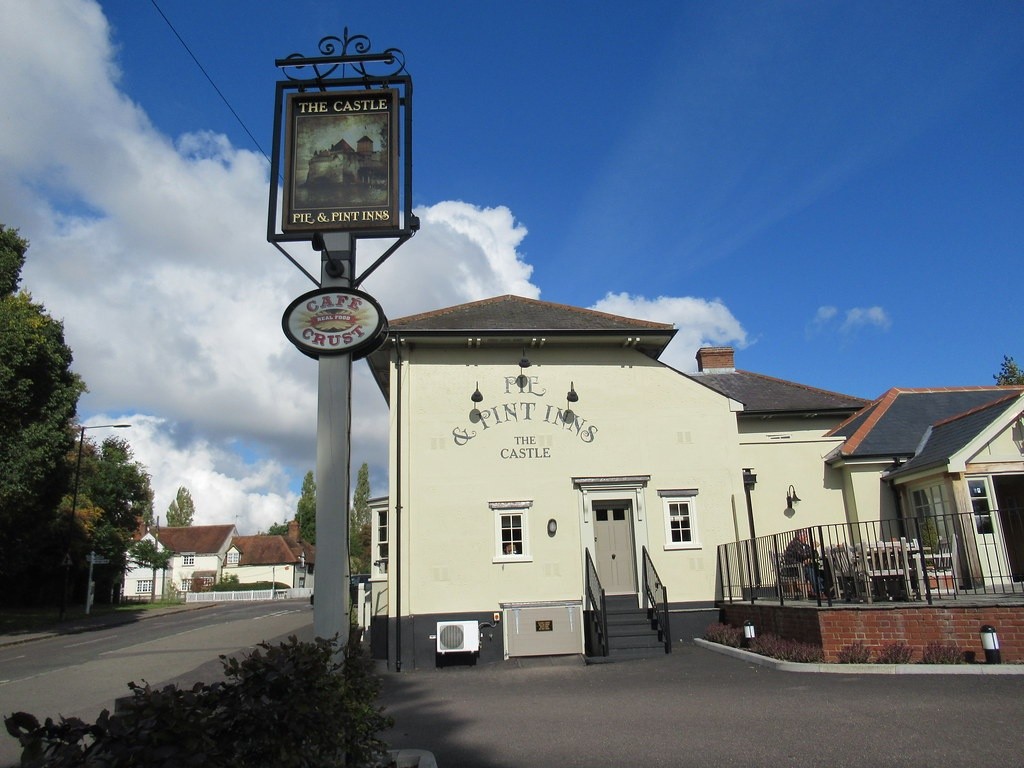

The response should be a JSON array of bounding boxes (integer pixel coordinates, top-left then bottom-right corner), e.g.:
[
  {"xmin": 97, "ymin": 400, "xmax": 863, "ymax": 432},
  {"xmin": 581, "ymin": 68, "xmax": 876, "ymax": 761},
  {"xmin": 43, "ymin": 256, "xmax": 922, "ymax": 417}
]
[{"xmin": 56, "ymin": 423, "xmax": 132, "ymax": 626}]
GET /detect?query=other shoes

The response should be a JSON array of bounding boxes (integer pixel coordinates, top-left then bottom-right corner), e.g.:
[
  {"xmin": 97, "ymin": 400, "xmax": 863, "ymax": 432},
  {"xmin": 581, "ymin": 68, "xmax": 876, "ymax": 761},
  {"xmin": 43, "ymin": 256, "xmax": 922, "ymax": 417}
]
[{"xmin": 814, "ymin": 591, "xmax": 832, "ymax": 599}]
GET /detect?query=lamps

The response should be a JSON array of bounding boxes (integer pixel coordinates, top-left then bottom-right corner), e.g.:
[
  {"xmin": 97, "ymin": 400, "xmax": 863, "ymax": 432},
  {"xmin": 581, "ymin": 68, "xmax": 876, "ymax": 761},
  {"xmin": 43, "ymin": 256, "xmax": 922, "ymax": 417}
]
[
  {"xmin": 518, "ymin": 346, "xmax": 531, "ymax": 378},
  {"xmin": 786, "ymin": 484, "xmax": 801, "ymax": 509},
  {"xmin": 471, "ymin": 380, "xmax": 484, "ymax": 402},
  {"xmin": 566, "ymin": 381, "xmax": 579, "ymax": 402}
]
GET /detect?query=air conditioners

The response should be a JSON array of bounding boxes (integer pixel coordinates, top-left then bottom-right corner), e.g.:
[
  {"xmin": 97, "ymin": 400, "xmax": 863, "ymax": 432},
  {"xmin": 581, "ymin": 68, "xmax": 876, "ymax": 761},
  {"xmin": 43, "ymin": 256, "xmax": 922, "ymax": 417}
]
[{"xmin": 436, "ymin": 619, "xmax": 480, "ymax": 656}]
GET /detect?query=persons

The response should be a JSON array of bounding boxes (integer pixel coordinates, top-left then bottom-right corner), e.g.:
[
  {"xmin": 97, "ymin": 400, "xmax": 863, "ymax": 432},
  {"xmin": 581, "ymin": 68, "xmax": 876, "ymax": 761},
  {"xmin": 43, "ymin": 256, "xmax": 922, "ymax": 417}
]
[{"xmin": 785, "ymin": 530, "xmax": 829, "ymax": 600}]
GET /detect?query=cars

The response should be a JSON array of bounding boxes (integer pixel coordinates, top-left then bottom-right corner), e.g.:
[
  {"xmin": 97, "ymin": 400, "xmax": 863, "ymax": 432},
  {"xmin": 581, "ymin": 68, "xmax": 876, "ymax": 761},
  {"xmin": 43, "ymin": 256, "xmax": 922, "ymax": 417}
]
[{"xmin": 310, "ymin": 574, "xmax": 373, "ymax": 606}]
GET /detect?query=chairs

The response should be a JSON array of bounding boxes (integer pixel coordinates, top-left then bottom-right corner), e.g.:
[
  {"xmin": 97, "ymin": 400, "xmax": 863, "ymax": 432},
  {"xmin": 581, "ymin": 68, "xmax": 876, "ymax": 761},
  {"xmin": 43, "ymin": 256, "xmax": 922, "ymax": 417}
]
[
  {"xmin": 823, "ymin": 532, "xmax": 959, "ymax": 600},
  {"xmin": 774, "ymin": 553, "xmax": 809, "ymax": 600}
]
